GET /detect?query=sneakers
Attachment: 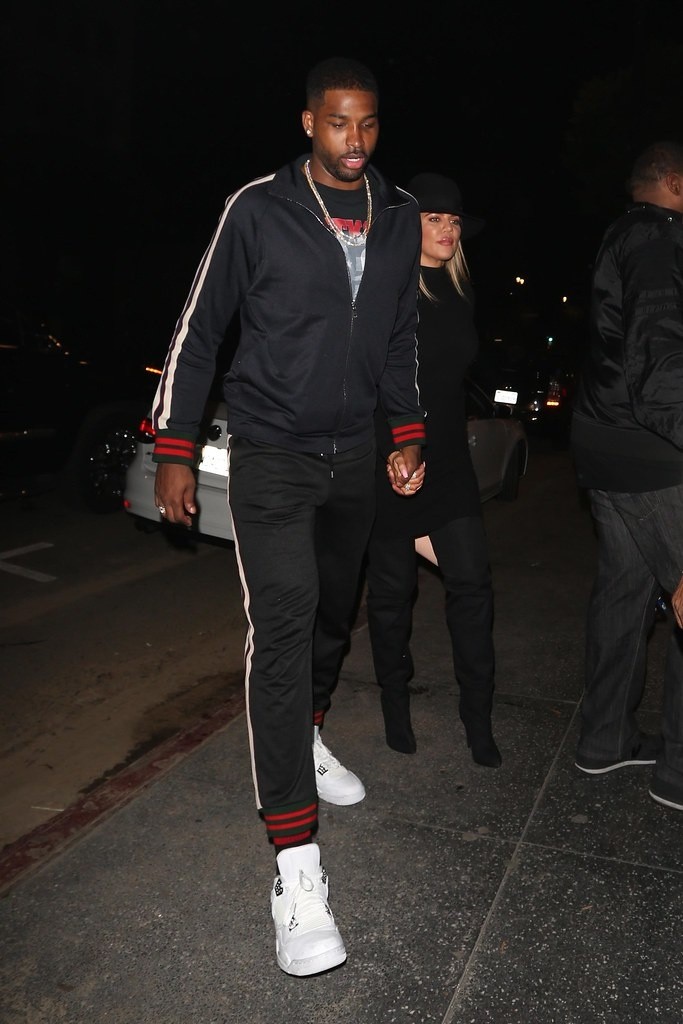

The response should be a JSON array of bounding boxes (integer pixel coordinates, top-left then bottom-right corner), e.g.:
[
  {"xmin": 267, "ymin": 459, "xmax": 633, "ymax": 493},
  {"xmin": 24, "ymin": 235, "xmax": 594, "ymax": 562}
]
[
  {"xmin": 574, "ymin": 731, "xmax": 670, "ymax": 775},
  {"xmin": 269, "ymin": 843, "xmax": 348, "ymax": 976},
  {"xmin": 313, "ymin": 723, "xmax": 367, "ymax": 806},
  {"xmin": 648, "ymin": 775, "xmax": 683, "ymax": 812}
]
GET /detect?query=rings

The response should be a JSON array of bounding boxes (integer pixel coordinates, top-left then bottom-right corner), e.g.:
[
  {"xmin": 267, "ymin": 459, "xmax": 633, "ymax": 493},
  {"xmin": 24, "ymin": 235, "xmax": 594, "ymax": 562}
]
[
  {"xmin": 405, "ymin": 482, "xmax": 410, "ymax": 491},
  {"xmin": 158, "ymin": 506, "xmax": 165, "ymax": 514},
  {"xmin": 412, "ymin": 472, "xmax": 417, "ymax": 479}
]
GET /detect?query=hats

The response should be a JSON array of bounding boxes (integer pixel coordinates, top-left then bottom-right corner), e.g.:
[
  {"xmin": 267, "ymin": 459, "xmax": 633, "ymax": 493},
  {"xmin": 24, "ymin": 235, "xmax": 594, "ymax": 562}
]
[{"xmin": 409, "ymin": 174, "xmax": 464, "ymax": 217}]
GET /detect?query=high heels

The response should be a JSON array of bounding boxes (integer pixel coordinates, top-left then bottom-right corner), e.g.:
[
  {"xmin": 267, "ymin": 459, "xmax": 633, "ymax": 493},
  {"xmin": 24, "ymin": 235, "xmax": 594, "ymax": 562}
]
[
  {"xmin": 381, "ymin": 688, "xmax": 418, "ymax": 755},
  {"xmin": 458, "ymin": 699, "xmax": 504, "ymax": 768}
]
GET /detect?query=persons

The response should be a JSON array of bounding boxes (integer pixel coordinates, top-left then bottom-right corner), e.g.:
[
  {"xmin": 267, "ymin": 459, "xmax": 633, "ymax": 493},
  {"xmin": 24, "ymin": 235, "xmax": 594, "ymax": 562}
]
[
  {"xmin": 368, "ymin": 169, "xmax": 506, "ymax": 771},
  {"xmin": 148, "ymin": 54, "xmax": 431, "ymax": 980},
  {"xmin": 671, "ymin": 573, "xmax": 683, "ymax": 631},
  {"xmin": 567, "ymin": 139, "xmax": 683, "ymax": 812}
]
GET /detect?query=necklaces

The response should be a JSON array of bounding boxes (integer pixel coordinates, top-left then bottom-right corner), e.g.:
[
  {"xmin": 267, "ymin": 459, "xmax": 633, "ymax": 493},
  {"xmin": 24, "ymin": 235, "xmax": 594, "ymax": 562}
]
[{"xmin": 301, "ymin": 159, "xmax": 375, "ymax": 248}]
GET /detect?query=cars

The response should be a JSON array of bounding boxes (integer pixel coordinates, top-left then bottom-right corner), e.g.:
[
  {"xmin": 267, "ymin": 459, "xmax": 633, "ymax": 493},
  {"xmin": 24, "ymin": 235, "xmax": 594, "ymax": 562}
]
[
  {"xmin": 117, "ymin": 378, "xmax": 534, "ymax": 557},
  {"xmin": 500, "ymin": 366, "xmax": 577, "ymax": 431}
]
